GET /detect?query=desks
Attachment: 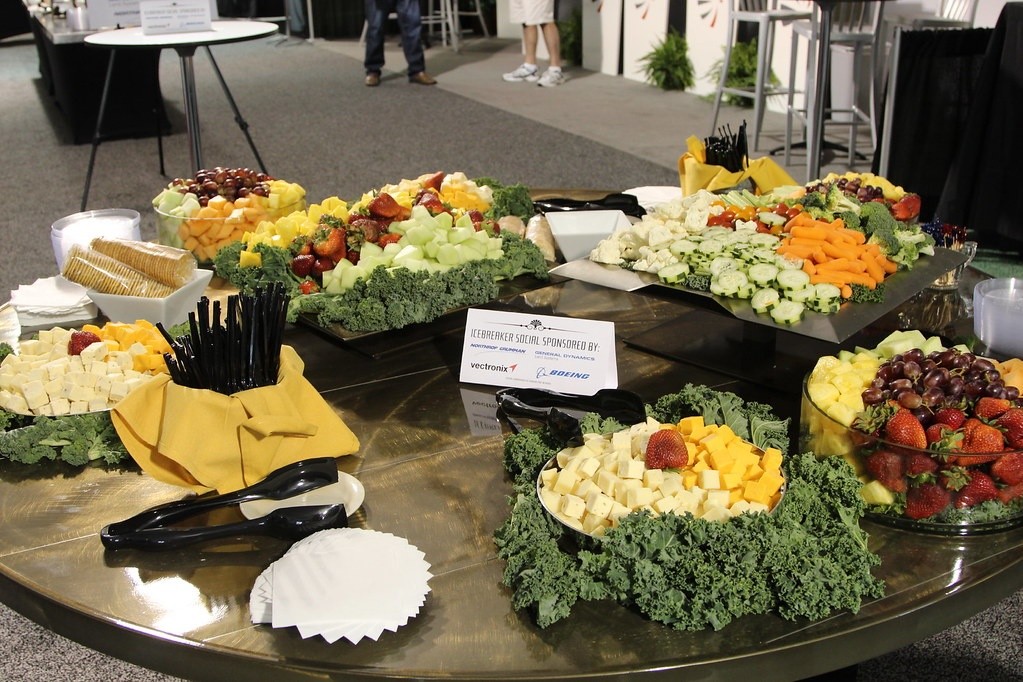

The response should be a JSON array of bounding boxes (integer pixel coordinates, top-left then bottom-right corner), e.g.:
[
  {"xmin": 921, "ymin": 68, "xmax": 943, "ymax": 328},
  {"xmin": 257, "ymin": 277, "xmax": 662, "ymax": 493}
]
[
  {"xmin": 80, "ymin": 20, "xmax": 279, "ymax": 213},
  {"xmin": 24, "ymin": 1, "xmax": 174, "ymax": 144}
]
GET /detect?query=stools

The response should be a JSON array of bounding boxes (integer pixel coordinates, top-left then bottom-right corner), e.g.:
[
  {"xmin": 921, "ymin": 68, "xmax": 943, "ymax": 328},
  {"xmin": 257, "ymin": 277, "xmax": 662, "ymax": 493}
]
[
  {"xmin": 708, "ymin": 0, "xmax": 817, "ymax": 169},
  {"xmin": 875, "ymin": 0, "xmax": 979, "ymax": 130},
  {"xmin": 783, "ymin": 0, "xmax": 886, "ymax": 168}
]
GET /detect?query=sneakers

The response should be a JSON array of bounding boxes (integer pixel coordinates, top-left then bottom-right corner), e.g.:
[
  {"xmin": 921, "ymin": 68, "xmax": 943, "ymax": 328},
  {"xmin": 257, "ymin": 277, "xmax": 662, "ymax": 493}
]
[
  {"xmin": 536, "ymin": 70, "xmax": 565, "ymax": 87},
  {"xmin": 502, "ymin": 65, "xmax": 539, "ymax": 83}
]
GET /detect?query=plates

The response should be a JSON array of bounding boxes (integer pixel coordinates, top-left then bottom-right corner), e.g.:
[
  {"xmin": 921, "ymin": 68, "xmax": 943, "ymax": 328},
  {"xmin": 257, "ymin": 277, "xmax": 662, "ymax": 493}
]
[
  {"xmin": 547, "ymin": 244, "xmax": 969, "ymax": 344},
  {"xmin": 544, "ymin": 209, "xmax": 635, "ymax": 262},
  {"xmin": 535, "ymin": 424, "xmax": 786, "ymax": 541},
  {"xmin": 239, "ymin": 471, "xmax": 365, "ymax": 522},
  {"xmin": 620, "ymin": 184, "xmax": 684, "ymax": 220},
  {"xmin": 15, "ymin": 406, "xmax": 116, "ymax": 417},
  {"xmin": 297, "ymin": 268, "xmax": 576, "ymax": 344}
]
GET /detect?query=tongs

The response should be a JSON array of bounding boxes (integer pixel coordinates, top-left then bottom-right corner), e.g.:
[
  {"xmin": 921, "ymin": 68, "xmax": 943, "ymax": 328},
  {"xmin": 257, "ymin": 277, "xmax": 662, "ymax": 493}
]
[
  {"xmin": 101, "ymin": 457, "xmax": 350, "ymax": 551},
  {"xmin": 530, "ymin": 193, "xmax": 648, "ymax": 220},
  {"xmin": 495, "ymin": 386, "xmax": 646, "ymax": 447}
]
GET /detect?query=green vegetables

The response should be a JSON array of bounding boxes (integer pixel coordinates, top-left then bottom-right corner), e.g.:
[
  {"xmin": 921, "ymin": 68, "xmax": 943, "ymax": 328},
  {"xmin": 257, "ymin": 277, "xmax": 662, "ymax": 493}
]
[
  {"xmin": 492, "ymin": 383, "xmax": 886, "ymax": 633},
  {"xmin": 212, "ymin": 178, "xmax": 550, "ymax": 331},
  {"xmin": 0, "ymin": 406, "xmax": 131, "ymax": 469}
]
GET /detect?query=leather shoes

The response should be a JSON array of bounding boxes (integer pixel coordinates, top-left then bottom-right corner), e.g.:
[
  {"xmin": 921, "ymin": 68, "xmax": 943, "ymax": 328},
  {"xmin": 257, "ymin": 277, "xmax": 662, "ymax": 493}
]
[
  {"xmin": 409, "ymin": 71, "xmax": 438, "ymax": 84},
  {"xmin": 365, "ymin": 73, "xmax": 380, "ymax": 85}
]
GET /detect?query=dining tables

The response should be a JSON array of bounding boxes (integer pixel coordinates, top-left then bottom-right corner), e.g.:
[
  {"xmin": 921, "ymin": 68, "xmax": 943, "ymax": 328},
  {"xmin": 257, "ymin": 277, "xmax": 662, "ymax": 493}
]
[{"xmin": 0, "ymin": 187, "xmax": 1023, "ymax": 682}]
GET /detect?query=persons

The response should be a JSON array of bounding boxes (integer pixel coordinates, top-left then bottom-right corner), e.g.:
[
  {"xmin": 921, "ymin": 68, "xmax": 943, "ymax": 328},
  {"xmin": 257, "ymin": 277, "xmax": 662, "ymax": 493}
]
[
  {"xmin": 361, "ymin": 1, "xmax": 437, "ymax": 87},
  {"xmin": 500, "ymin": 1, "xmax": 565, "ymax": 87}
]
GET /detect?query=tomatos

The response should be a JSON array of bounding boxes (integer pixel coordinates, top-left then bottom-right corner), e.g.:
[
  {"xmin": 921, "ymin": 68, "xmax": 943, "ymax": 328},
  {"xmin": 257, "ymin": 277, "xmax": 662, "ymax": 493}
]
[{"xmin": 708, "ymin": 200, "xmax": 804, "ymax": 237}]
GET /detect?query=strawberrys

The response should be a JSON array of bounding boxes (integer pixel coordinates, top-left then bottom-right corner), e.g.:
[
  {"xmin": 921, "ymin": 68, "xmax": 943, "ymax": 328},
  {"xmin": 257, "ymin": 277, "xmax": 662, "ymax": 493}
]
[
  {"xmin": 645, "ymin": 429, "xmax": 689, "ymax": 469},
  {"xmin": 67, "ymin": 331, "xmax": 101, "ymax": 355},
  {"xmin": 287, "ymin": 171, "xmax": 501, "ymax": 295},
  {"xmin": 850, "ymin": 396, "xmax": 1023, "ymax": 520}
]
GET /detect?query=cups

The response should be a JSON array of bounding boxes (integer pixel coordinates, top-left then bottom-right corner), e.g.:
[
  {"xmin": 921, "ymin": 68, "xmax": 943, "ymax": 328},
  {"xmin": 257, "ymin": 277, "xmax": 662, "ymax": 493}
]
[
  {"xmin": 49, "ymin": 207, "xmax": 141, "ymax": 275},
  {"xmin": 927, "ymin": 234, "xmax": 979, "ymax": 289}
]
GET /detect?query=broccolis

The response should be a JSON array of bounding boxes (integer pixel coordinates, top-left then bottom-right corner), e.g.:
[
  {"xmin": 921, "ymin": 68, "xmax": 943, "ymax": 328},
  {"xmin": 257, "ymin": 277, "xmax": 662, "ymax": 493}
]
[{"xmin": 793, "ymin": 182, "xmax": 936, "ymax": 271}]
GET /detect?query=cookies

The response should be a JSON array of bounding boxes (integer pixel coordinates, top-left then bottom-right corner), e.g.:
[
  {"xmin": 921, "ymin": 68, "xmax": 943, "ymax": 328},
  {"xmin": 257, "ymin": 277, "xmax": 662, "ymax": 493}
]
[{"xmin": 61, "ymin": 235, "xmax": 194, "ymax": 298}]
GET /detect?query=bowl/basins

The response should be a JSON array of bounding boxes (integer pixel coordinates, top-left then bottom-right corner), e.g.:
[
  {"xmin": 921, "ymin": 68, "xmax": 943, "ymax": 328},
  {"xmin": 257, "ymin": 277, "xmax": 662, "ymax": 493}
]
[
  {"xmin": 153, "ymin": 195, "xmax": 306, "ymax": 267},
  {"xmin": 87, "ymin": 268, "xmax": 213, "ymax": 329},
  {"xmin": 798, "ymin": 366, "xmax": 1023, "ymax": 537},
  {"xmin": 974, "ymin": 277, "xmax": 1022, "ymax": 357}
]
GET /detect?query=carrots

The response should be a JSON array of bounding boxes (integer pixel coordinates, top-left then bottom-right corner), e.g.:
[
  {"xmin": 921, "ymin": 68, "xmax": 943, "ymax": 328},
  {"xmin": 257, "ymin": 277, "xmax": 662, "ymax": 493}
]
[{"xmin": 776, "ymin": 212, "xmax": 898, "ymax": 299}]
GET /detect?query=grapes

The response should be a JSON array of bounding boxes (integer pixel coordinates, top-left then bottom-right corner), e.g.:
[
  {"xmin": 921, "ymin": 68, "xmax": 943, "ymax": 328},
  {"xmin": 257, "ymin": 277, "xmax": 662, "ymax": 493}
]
[
  {"xmin": 172, "ymin": 166, "xmax": 274, "ymax": 207},
  {"xmin": 861, "ymin": 348, "xmax": 1023, "ymax": 423},
  {"xmin": 805, "ymin": 178, "xmax": 884, "ymax": 203}
]
